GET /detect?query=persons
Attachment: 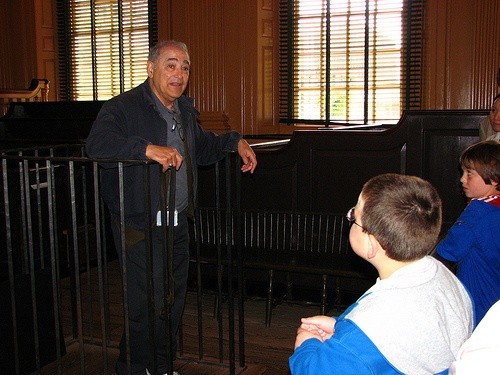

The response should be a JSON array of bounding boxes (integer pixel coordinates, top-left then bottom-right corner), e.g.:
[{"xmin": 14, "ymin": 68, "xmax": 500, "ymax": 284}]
[
  {"xmin": 289, "ymin": 173, "xmax": 475, "ymax": 375},
  {"xmin": 480, "ymin": 94, "xmax": 500, "ymax": 145},
  {"xmin": 435, "ymin": 141, "xmax": 500, "ymax": 326},
  {"xmin": 86, "ymin": 41, "xmax": 259, "ymax": 374}
]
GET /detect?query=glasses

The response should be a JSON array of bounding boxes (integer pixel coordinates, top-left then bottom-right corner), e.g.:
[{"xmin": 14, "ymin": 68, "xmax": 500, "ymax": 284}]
[
  {"xmin": 168, "ymin": 109, "xmax": 186, "ymax": 142},
  {"xmin": 346, "ymin": 207, "xmax": 373, "ymax": 236}
]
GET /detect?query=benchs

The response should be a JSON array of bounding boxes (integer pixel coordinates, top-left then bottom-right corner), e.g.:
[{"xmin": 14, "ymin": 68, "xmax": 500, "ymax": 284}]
[{"xmin": 188, "ymin": 207, "xmax": 457, "ymax": 328}]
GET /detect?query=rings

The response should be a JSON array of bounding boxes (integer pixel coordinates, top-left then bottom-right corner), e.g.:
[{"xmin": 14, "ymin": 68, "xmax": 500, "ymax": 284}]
[{"xmin": 168, "ymin": 164, "xmax": 173, "ymax": 166}]
